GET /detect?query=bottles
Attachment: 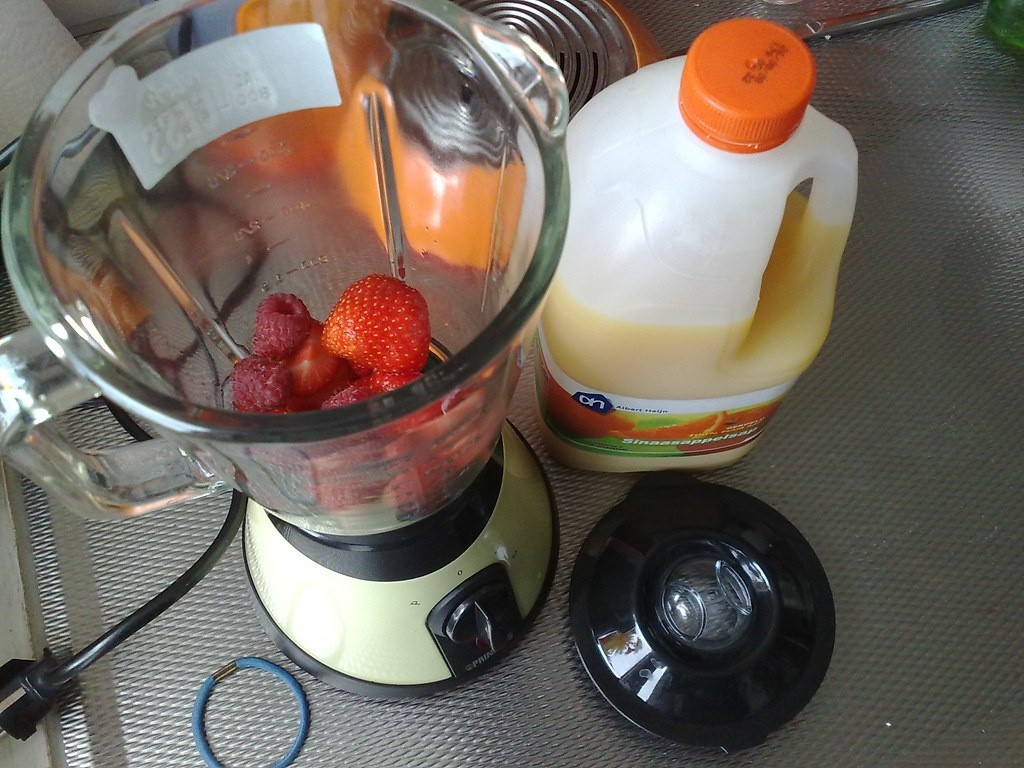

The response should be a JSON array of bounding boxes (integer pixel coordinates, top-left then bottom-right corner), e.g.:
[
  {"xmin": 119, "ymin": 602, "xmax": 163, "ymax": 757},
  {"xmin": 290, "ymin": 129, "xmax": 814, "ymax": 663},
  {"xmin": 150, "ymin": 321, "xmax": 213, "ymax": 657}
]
[{"xmin": 532, "ymin": 17, "xmax": 859, "ymax": 475}]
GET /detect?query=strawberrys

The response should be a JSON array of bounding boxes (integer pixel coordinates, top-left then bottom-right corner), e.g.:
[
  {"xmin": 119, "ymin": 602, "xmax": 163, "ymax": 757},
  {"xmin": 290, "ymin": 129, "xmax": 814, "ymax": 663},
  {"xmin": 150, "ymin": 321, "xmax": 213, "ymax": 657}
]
[{"xmin": 282, "ymin": 272, "xmax": 494, "ymax": 506}]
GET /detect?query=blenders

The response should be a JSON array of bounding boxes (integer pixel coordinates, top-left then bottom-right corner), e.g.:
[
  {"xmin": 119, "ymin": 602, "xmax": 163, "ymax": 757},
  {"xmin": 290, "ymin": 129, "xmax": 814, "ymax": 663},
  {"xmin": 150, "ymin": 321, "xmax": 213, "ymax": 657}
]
[{"xmin": 0, "ymin": 1, "xmax": 560, "ymax": 701}]
